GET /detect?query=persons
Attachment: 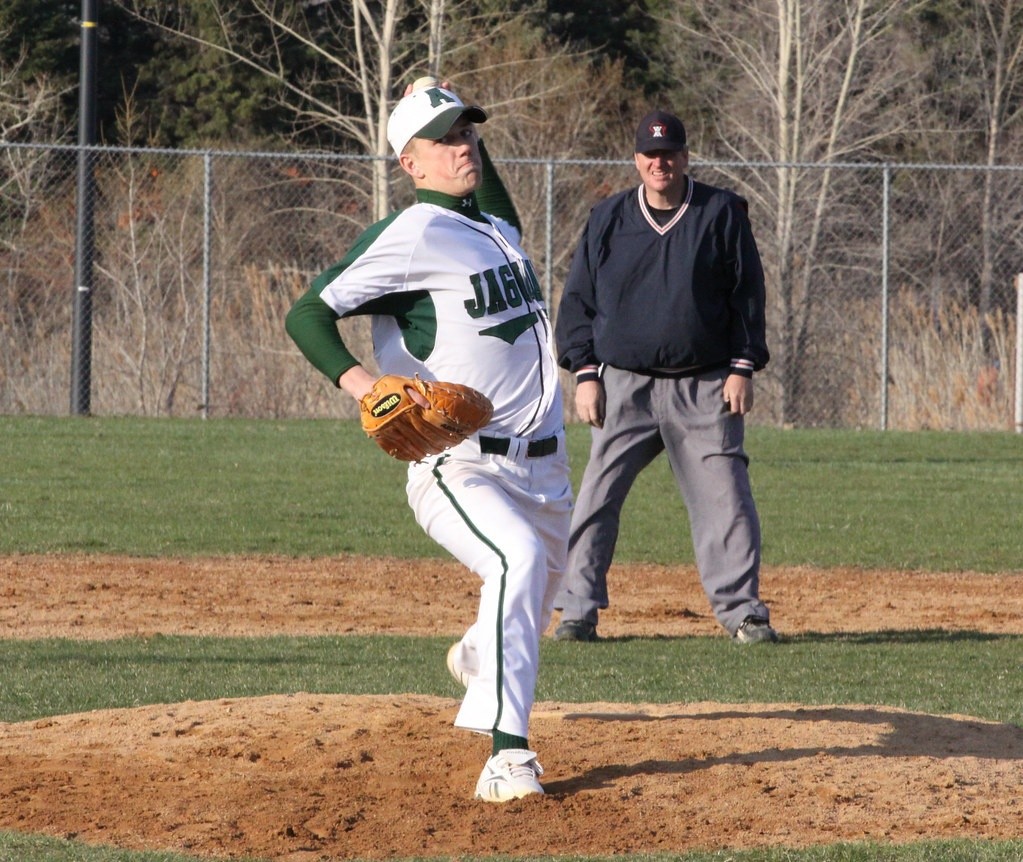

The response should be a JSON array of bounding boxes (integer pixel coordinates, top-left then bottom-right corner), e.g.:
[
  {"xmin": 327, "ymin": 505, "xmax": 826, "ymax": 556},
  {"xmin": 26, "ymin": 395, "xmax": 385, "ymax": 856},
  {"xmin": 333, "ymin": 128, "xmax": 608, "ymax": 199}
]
[
  {"xmin": 555, "ymin": 113, "xmax": 779, "ymax": 646},
  {"xmin": 284, "ymin": 83, "xmax": 575, "ymax": 803}
]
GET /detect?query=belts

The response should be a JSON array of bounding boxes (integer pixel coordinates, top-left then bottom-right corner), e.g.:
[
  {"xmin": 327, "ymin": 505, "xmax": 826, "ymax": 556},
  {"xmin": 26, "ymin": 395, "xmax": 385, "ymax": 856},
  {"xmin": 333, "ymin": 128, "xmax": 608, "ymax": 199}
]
[{"xmin": 479, "ymin": 426, "xmax": 564, "ymax": 457}]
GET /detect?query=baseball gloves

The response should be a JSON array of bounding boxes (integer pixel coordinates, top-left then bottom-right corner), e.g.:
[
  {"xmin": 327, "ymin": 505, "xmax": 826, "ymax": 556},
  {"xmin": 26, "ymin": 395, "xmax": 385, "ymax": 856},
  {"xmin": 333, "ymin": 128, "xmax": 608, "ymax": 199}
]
[{"xmin": 356, "ymin": 371, "xmax": 497, "ymax": 470}]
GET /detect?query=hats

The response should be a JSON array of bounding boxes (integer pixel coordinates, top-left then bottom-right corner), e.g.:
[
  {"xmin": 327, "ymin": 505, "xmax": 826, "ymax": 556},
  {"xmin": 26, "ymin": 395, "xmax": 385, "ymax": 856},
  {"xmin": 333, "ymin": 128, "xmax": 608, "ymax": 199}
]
[
  {"xmin": 636, "ymin": 110, "xmax": 686, "ymax": 153},
  {"xmin": 387, "ymin": 87, "xmax": 488, "ymax": 159}
]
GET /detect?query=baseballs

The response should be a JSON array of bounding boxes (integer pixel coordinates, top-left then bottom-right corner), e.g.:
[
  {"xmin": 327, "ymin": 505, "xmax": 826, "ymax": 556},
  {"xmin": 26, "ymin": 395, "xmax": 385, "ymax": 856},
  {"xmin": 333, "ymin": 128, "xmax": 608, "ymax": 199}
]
[{"xmin": 412, "ymin": 76, "xmax": 441, "ymax": 94}]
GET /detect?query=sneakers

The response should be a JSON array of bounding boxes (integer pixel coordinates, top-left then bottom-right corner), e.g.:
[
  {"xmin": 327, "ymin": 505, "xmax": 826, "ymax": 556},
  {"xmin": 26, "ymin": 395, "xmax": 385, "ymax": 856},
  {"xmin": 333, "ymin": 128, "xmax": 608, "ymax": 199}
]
[
  {"xmin": 735, "ymin": 615, "xmax": 784, "ymax": 644},
  {"xmin": 555, "ymin": 620, "xmax": 597, "ymax": 641},
  {"xmin": 475, "ymin": 749, "xmax": 544, "ymax": 803},
  {"xmin": 446, "ymin": 642, "xmax": 478, "ymax": 689}
]
